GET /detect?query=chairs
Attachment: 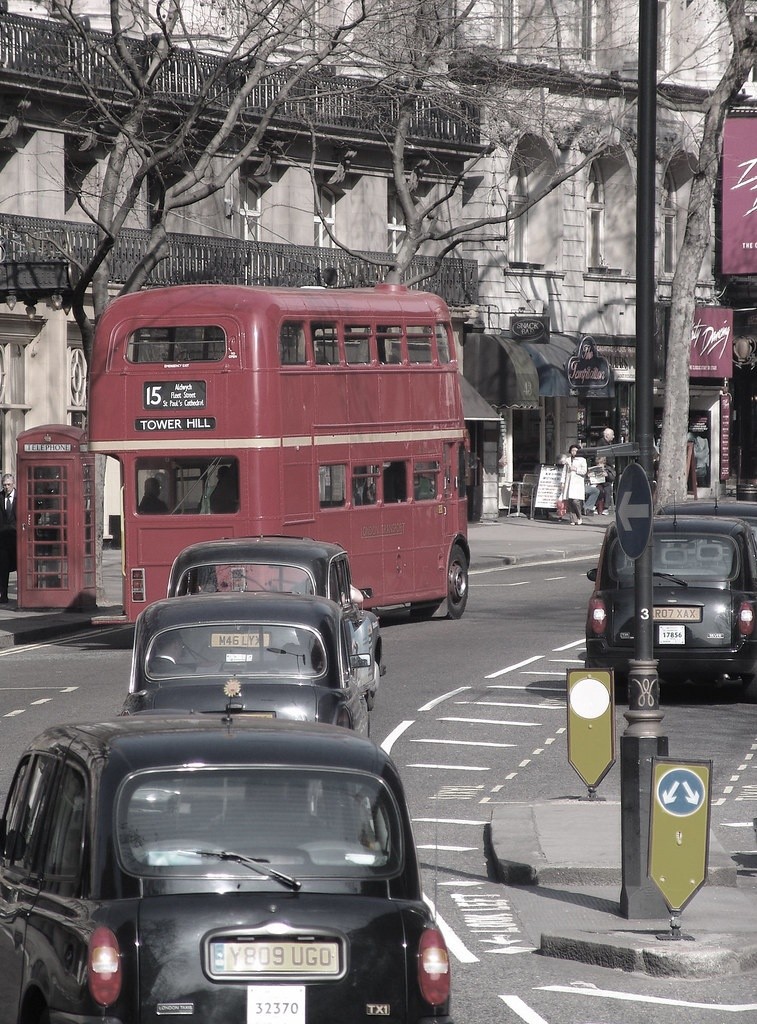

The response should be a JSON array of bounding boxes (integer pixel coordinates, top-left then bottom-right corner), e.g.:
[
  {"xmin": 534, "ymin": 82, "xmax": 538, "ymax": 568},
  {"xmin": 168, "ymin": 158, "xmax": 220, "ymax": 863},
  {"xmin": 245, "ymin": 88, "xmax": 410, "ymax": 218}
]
[{"xmin": 506, "ymin": 474, "xmax": 541, "ymax": 520}]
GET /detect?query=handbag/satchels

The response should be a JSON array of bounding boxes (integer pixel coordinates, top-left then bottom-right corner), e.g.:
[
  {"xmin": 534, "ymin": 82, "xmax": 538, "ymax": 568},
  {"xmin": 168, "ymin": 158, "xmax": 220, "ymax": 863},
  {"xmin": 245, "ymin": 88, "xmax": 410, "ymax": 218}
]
[{"xmin": 557, "ymin": 488, "xmax": 567, "ymax": 516}]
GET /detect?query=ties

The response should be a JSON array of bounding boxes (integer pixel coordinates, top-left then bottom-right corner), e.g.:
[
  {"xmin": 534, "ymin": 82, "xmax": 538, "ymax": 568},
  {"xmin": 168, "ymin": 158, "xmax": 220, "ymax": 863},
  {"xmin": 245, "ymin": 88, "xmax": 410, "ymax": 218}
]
[{"xmin": 7, "ymin": 495, "xmax": 11, "ymax": 513}]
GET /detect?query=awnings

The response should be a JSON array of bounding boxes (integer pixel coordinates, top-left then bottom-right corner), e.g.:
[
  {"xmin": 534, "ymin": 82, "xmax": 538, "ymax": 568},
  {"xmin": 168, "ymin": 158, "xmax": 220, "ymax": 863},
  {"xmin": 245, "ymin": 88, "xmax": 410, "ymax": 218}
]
[
  {"xmin": 523, "ymin": 336, "xmax": 616, "ymax": 398},
  {"xmin": 317, "ymin": 340, "xmax": 505, "ymax": 422},
  {"xmin": 463, "ymin": 334, "xmax": 540, "ymax": 410}
]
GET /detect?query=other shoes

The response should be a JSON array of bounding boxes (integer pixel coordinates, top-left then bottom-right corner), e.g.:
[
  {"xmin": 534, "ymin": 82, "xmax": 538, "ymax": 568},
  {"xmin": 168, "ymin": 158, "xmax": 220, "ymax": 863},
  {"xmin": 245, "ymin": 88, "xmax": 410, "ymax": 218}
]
[
  {"xmin": 592, "ymin": 509, "xmax": 599, "ymax": 515},
  {"xmin": 602, "ymin": 509, "xmax": 609, "ymax": 515},
  {"xmin": 0, "ymin": 596, "xmax": 8, "ymax": 603},
  {"xmin": 570, "ymin": 522, "xmax": 575, "ymax": 525},
  {"xmin": 582, "ymin": 505, "xmax": 588, "ymax": 516},
  {"xmin": 577, "ymin": 517, "xmax": 582, "ymax": 525}
]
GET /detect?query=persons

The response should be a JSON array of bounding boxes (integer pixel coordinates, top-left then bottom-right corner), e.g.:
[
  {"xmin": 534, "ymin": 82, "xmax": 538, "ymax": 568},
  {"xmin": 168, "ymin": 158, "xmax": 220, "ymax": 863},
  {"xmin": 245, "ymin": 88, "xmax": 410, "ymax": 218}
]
[
  {"xmin": 137, "ymin": 477, "xmax": 169, "ymax": 515},
  {"xmin": 557, "ymin": 427, "xmax": 616, "ymax": 525},
  {"xmin": 210, "ymin": 465, "xmax": 237, "ymax": 513},
  {"xmin": 0, "ymin": 474, "xmax": 19, "ymax": 604}
]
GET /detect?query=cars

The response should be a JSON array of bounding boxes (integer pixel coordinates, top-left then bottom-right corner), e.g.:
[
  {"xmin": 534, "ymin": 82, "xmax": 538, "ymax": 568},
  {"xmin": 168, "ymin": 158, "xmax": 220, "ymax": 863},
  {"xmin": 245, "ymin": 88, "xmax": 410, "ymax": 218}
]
[
  {"xmin": 585, "ymin": 496, "xmax": 757, "ymax": 702},
  {"xmin": 116, "ymin": 536, "xmax": 388, "ymax": 735},
  {"xmin": 0, "ymin": 707, "xmax": 452, "ymax": 1024}
]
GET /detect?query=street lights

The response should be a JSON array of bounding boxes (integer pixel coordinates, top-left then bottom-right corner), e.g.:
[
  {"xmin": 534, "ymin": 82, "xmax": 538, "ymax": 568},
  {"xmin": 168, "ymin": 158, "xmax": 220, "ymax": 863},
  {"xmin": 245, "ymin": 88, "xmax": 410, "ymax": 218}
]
[{"xmin": 725, "ymin": 334, "xmax": 757, "ymax": 486}]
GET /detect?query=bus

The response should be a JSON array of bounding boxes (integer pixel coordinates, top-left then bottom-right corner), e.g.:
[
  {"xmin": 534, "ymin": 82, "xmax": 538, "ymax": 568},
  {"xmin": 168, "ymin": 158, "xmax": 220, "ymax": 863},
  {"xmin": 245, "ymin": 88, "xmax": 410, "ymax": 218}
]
[{"xmin": 87, "ymin": 281, "xmax": 473, "ymax": 633}]
